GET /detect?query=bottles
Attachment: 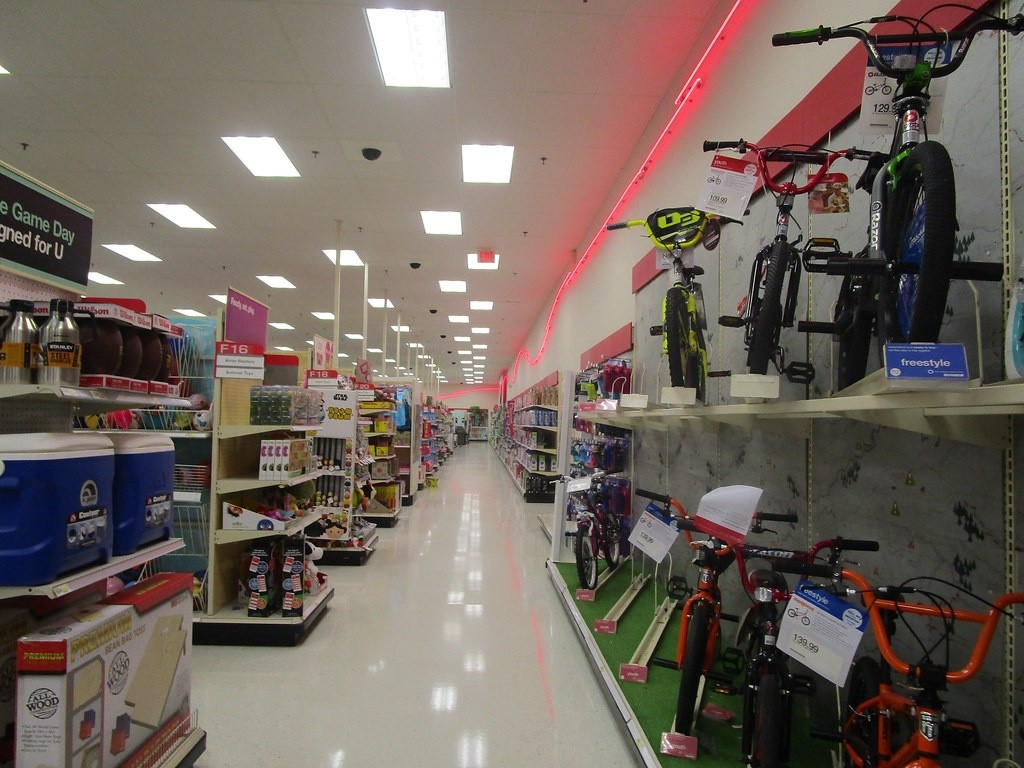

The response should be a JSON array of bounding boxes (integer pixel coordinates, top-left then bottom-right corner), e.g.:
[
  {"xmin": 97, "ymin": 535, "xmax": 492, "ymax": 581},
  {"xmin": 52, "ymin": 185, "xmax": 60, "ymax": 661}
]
[
  {"xmin": 249, "ymin": 386, "xmax": 320, "ymax": 425},
  {"xmin": 0, "ymin": 299, "xmax": 39, "ymax": 386},
  {"xmin": 39, "ymin": 299, "xmax": 97, "ymax": 387}
]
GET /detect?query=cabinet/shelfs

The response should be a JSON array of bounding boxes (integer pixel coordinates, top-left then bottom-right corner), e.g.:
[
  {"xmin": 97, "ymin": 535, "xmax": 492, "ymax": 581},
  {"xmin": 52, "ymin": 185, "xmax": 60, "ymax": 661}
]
[
  {"xmin": 178, "ymin": 309, "xmax": 334, "ymax": 644},
  {"xmin": 0, "ymin": 308, "xmax": 206, "ymax": 768},
  {"xmin": 305, "ymin": 362, "xmax": 379, "ymax": 567},
  {"xmin": 379, "ymin": 374, "xmax": 420, "ymax": 510},
  {"xmin": 489, "ymin": 371, "xmax": 563, "ymax": 503},
  {"xmin": 341, "ymin": 383, "xmax": 402, "ymax": 527},
  {"xmin": 420, "ymin": 377, "xmax": 453, "ymax": 508}
]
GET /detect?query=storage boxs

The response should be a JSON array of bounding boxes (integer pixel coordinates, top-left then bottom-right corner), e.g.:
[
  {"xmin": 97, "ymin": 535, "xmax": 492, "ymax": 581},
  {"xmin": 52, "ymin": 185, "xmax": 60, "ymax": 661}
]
[
  {"xmin": 249, "ymin": 385, "xmax": 322, "ymax": 426},
  {"xmin": 259, "ymin": 439, "xmax": 318, "ymax": 481},
  {"xmin": 372, "ymin": 462, "xmax": 393, "ymax": 480},
  {"xmin": 15, "ymin": 573, "xmax": 197, "ymax": 768},
  {"xmin": 376, "ymin": 442, "xmax": 389, "ymax": 456},
  {"xmin": 364, "ymin": 417, "xmax": 397, "ymax": 433},
  {"xmin": 108, "ymin": 433, "xmax": 176, "ymax": 556},
  {"xmin": 375, "ymin": 484, "xmax": 399, "ymax": 512},
  {"xmin": 0, "ymin": 431, "xmax": 115, "ymax": 587},
  {"xmin": 512, "ymin": 411, "xmax": 555, "ymax": 489}
]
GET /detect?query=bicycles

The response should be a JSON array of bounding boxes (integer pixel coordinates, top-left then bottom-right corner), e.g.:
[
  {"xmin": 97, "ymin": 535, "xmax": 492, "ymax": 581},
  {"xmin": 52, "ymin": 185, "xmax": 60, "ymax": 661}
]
[
  {"xmin": 772, "ymin": 557, "xmax": 1024, "ymax": 768},
  {"xmin": 704, "ymin": 138, "xmax": 869, "ymax": 384},
  {"xmin": 634, "ymin": 489, "xmax": 880, "ymax": 768},
  {"xmin": 773, "ymin": 3, "xmax": 1024, "ymax": 392},
  {"xmin": 607, "ymin": 206, "xmax": 743, "ymax": 403},
  {"xmin": 549, "ymin": 473, "xmax": 628, "ymax": 590}
]
